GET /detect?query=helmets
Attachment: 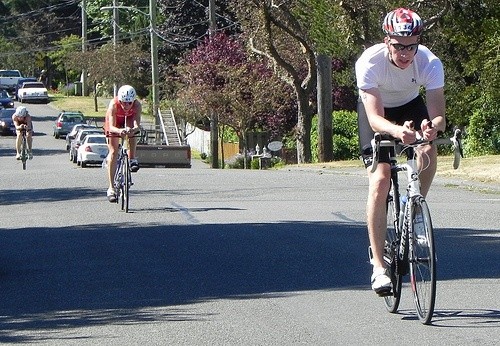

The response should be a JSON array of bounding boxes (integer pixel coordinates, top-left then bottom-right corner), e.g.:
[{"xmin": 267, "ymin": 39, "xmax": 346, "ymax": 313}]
[
  {"xmin": 15, "ymin": 106, "xmax": 28, "ymax": 118},
  {"xmin": 117, "ymin": 85, "xmax": 136, "ymax": 103},
  {"xmin": 382, "ymin": 8, "xmax": 423, "ymax": 37}
]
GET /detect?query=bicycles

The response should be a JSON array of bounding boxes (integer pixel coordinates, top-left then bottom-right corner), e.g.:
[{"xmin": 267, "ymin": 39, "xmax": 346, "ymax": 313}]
[
  {"xmin": 106, "ymin": 128, "xmax": 147, "ymax": 213},
  {"xmin": 367, "ymin": 120, "xmax": 464, "ymax": 327},
  {"xmin": 17, "ymin": 127, "xmax": 31, "ymax": 171}
]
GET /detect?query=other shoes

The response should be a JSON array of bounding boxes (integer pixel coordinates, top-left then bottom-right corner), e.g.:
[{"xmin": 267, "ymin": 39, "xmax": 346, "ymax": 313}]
[
  {"xmin": 106, "ymin": 189, "xmax": 117, "ymax": 203},
  {"xmin": 130, "ymin": 159, "xmax": 140, "ymax": 172},
  {"xmin": 370, "ymin": 268, "xmax": 392, "ymax": 293},
  {"xmin": 28, "ymin": 152, "xmax": 33, "ymax": 160},
  {"xmin": 15, "ymin": 149, "xmax": 21, "ymax": 159},
  {"xmin": 401, "ymin": 196, "xmax": 429, "ymax": 222}
]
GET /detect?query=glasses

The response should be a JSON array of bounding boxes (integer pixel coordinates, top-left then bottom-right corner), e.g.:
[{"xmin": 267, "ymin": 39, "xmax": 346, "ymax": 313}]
[
  {"xmin": 122, "ymin": 102, "xmax": 133, "ymax": 105},
  {"xmin": 387, "ymin": 38, "xmax": 419, "ymax": 52}
]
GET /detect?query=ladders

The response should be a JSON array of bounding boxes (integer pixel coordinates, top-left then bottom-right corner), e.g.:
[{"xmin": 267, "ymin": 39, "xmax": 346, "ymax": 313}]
[{"xmin": 158, "ymin": 108, "xmax": 182, "ymax": 145}]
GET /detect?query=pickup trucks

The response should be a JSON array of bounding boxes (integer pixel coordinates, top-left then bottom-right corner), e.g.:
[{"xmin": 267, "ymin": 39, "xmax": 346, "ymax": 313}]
[{"xmin": 0, "ymin": 70, "xmax": 23, "ymax": 93}]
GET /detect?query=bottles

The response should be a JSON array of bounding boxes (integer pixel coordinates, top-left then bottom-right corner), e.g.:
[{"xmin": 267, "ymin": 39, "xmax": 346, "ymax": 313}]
[{"xmin": 398, "ymin": 194, "xmax": 409, "ymax": 233}]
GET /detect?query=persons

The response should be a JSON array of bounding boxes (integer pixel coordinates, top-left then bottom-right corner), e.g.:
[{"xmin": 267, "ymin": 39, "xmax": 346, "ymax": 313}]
[
  {"xmin": 12, "ymin": 107, "xmax": 33, "ymax": 160},
  {"xmin": 356, "ymin": 8, "xmax": 447, "ymax": 292},
  {"xmin": 104, "ymin": 84, "xmax": 142, "ymax": 203}
]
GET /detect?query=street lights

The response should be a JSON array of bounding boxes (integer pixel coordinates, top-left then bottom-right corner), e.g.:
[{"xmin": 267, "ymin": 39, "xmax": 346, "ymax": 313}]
[{"xmin": 99, "ymin": 6, "xmax": 161, "ymax": 142}]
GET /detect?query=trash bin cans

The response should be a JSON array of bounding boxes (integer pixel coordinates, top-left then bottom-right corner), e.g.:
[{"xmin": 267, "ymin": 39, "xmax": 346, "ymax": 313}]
[{"xmin": 73, "ymin": 80, "xmax": 82, "ymax": 96}]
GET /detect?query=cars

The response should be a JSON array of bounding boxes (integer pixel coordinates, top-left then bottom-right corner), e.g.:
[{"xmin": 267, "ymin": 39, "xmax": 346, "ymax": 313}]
[
  {"xmin": 65, "ymin": 123, "xmax": 106, "ymax": 164},
  {"xmin": 0, "ymin": 108, "xmax": 17, "ymax": 136},
  {"xmin": 0, "ymin": 89, "xmax": 14, "ymax": 110},
  {"xmin": 75, "ymin": 134, "xmax": 109, "ymax": 168},
  {"xmin": 17, "ymin": 81, "xmax": 48, "ymax": 103}
]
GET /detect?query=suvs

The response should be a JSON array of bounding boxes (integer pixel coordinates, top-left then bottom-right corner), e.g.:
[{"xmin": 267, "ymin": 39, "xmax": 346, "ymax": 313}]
[
  {"xmin": 13, "ymin": 77, "xmax": 37, "ymax": 100},
  {"xmin": 53, "ymin": 110, "xmax": 87, "ymax": 139}
]
